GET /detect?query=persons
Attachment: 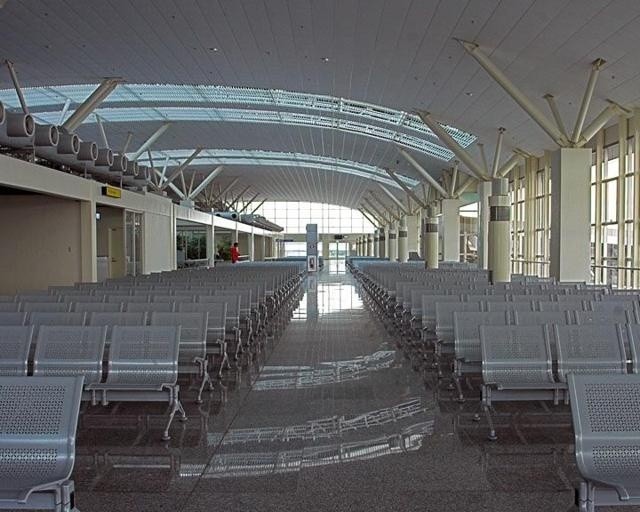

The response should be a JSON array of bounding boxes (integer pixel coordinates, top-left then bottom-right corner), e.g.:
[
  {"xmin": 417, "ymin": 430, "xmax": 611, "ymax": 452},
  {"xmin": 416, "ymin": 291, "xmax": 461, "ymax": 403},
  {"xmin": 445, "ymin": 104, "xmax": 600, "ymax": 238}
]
[{"xmin": 230, "ymin": 243, "xmax": 241, "ymax": 263}]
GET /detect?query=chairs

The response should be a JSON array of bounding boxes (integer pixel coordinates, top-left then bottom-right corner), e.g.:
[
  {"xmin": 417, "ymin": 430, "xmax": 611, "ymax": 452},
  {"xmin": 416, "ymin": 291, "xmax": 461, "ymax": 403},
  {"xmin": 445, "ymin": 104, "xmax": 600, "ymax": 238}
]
[
  {"xmin": 341, "ymin": 252, "xmax": 638, "ymax": 511},
  {"xmin": 0, "ymin": 254, "xmax": 309, "ymax": 511}
]
